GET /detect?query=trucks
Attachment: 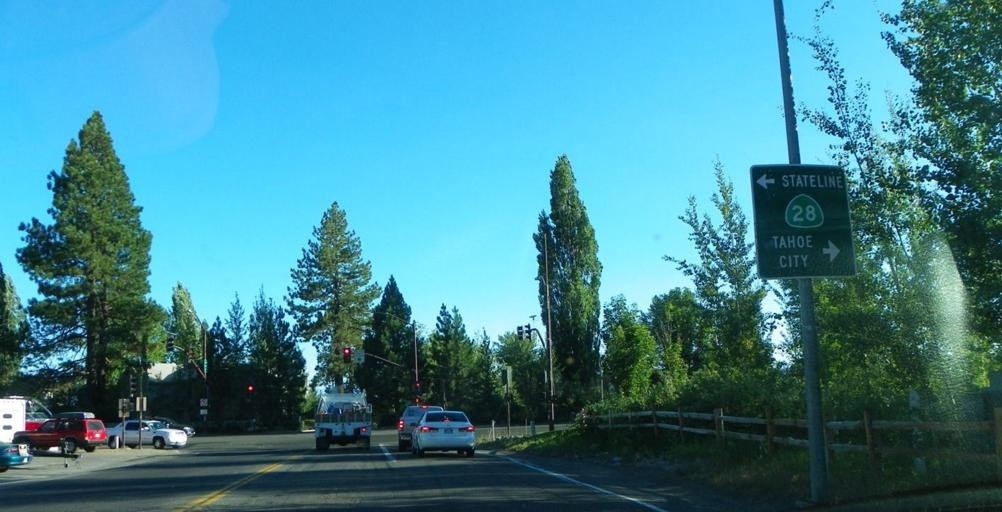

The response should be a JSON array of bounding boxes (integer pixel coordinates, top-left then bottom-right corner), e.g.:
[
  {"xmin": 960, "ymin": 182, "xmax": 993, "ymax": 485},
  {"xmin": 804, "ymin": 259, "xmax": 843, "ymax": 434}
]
[{"xmin": 312, "ymin": 388, "xmax": 374, "ymax": 451}]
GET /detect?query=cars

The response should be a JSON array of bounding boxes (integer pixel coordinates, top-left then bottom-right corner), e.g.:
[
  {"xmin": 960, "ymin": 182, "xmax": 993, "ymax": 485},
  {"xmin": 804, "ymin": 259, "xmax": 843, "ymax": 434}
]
[
  {"xmin": 397, "ymin": 404, "xmax": 444, "ymax": 452},
  {"xmin": 0, "ymin": 397, "xmax": 197, "ymax": 472},
  {"xmin": 411, "ymin": 410, "xmax": 476, "ymax": 458}
]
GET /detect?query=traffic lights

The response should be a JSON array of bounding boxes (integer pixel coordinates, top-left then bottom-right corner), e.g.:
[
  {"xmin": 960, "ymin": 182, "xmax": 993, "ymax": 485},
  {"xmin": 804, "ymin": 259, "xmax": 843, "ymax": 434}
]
[
  {"xmin": 517, "ymin": 326, "xmax": 524, "ymax": 341},
  {"xmin": 415, "ymin": 396, "xmax": 420, "ymax": 404},
  {"xmin": 524, "ymin": 323, "xmax": 532, "ymax": 342},
  {"xmin": 343, "ymin": 348, "xmax": 351, "ymax": 363},
  {"xmin": 416, "ymin": 381, "xmax": 420, "ymax": 390},
  {"xmin": 187, "ymin": 344, "xmax": 195, "ymax": 364},
  {"xmin": 165, "ymin": 333, "xmax": 174, "ymax": 352},
  {"xmin": 247, "ymin": 385, "xmax": 254, "ymax": 401},
  {"xmin": 128, "ymin": 373, "xmax": 138, "ymax": 397}
]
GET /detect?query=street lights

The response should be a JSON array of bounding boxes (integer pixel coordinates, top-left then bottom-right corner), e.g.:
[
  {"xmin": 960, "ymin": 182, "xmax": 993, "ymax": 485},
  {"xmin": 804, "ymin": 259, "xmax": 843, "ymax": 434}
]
[
  {"xmin": 187, "ymin": 308, "xmax": 211, "ymax": 435},
  {"xmin": 362, "ymin": 311, "xmax": 419, "ymax": 406}
]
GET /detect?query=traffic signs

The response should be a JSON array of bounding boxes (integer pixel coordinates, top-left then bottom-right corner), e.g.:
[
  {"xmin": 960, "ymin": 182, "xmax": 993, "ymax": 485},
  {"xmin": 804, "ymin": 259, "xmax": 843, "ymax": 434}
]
[{"xmin": 749, "ymin": 162, "xmax": 859, "ymax": 281}]
